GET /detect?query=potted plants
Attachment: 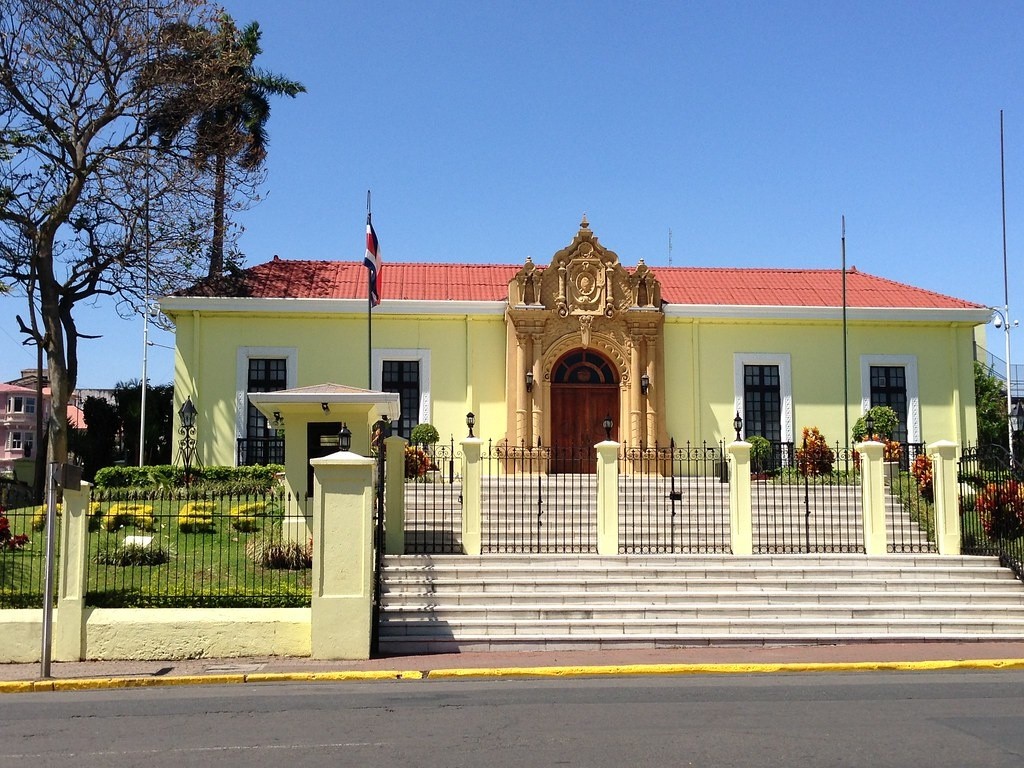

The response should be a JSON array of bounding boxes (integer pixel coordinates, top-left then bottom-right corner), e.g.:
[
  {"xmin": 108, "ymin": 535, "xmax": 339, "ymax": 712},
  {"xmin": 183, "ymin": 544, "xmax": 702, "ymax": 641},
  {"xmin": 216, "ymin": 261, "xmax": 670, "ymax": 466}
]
[{"xmin": 410, "ymin": 423, "xmax": 441, "ymax": 484}]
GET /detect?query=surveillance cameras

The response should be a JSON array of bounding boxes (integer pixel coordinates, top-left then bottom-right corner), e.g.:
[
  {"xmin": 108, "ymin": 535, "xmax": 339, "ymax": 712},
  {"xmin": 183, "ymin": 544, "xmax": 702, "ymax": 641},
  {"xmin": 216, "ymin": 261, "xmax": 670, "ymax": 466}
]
[{"xmin": 994, "ymin": 317, "xmax": 1002, "ymax": 328}]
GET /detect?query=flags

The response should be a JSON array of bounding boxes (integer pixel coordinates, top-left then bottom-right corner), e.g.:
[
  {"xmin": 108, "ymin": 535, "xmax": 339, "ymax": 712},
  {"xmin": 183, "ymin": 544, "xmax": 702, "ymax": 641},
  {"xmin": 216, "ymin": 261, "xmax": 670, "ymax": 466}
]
[{"xmin": 363, "ymin": 200, "xmax": 382, "ymax": 308}]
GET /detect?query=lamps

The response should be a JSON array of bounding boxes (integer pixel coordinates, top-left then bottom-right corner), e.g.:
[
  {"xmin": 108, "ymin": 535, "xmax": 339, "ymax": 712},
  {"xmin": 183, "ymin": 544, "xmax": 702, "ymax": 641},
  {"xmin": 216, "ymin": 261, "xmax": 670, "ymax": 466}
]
[
  {"xmin": 525, "ymin": 369, "xmax": 536, "ymax": 393},
  {"xmin": 177, "ymin": 396, "xmax": 197, "ymax": 428},
  {"xmin": 733, "ymin": 411, "xmax": 744, "ymax": 441},
  {"xmin": 321, "ymin": 403, "xmax": 331, "ymax": 416},
  {"xmin": 382, "ymin": 415, "xmax": 392, "ymax": 424},
  {"xmin": 641, "ymin": 371, "xmax": 650, "ymax": 396},
  {"xmin": 337, "ymin": 422, "xmax": 353, "ymax": 451},
  {"xmin": 271, "ymin": 412, "xmax": 285, "ymax": 428},
  {"xmin": 466, "ymin": 412, "xmax": 476, "ymax": 438},
  {"xmin": 602, "ymin": 412, "xmax": 614, "ymax": 441}
]
